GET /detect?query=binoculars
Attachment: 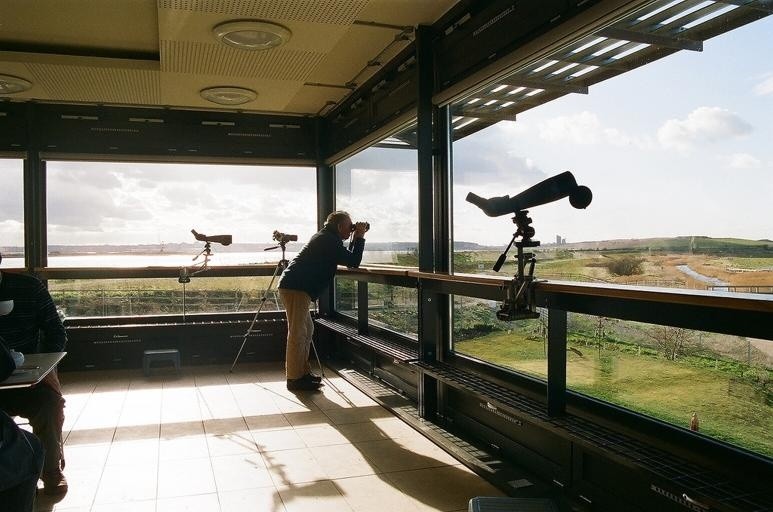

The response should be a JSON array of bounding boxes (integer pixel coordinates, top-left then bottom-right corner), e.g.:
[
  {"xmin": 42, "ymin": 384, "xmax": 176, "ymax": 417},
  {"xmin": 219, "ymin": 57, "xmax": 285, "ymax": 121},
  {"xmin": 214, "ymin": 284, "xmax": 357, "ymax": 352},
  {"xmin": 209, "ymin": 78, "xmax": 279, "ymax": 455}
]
[
  {"xmin": 465, "ymin": 171, "xmax": 593, "ymax": 217},
  {"xmin": 263, "ymin": 230, "xmax": 297, "ymax": 251},
  {"xmin": 351, "ymin": 224, "xmax": 369, "ymax": 231},
  {"xmin": 191, "ymin": 229, "xmax": 232, "ymax": 261}
]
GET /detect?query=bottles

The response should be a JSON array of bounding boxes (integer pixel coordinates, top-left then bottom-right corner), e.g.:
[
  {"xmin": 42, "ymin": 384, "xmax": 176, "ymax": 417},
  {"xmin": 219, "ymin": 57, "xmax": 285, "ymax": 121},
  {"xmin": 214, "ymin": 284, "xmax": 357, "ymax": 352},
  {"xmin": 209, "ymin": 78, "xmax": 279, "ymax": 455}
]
[
  {"xmin": 9, "ymin": 349, "xmax": 25, "ymax": 367},
  {"xmin": 691, "ymin": 413, "xmax": 699, "ymax": 431}
]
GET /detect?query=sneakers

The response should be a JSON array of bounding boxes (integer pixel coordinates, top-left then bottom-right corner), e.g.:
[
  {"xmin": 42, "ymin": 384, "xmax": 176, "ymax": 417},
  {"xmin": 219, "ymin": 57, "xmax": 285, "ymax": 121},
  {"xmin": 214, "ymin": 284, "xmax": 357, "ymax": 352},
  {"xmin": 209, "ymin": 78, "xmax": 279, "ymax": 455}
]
[
  {"xmin": 39, "ymin": 462, "xmax": 68, "ymax": 493},
  {"xmin": 286, "ymin": 374, "xmax": 322, "ymax": 390}
]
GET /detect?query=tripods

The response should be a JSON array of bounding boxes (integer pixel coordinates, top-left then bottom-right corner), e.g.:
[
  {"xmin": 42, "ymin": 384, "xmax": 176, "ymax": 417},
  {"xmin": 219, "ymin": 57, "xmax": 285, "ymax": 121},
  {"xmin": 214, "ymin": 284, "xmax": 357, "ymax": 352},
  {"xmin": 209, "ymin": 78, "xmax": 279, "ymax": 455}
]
[{"xmin": 229, "ymin": 260, "xmax": 325, "ymax": 379}]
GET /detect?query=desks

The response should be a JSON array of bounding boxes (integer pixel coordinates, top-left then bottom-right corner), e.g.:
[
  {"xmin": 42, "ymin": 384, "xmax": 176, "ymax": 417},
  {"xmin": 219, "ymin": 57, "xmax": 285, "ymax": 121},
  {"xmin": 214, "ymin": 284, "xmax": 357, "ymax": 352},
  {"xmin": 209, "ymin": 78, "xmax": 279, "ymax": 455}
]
[{"xmin": 0, "ymin": 351, "xmax": 68, "ymax": 497}]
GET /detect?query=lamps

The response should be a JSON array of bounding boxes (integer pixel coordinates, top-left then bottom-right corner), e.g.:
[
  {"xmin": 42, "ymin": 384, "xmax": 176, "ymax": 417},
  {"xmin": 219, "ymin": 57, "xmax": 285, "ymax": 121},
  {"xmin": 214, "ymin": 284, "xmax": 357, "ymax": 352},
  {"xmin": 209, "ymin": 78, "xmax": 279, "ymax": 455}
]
[
  {"xmin": 0, "ymin": 73, "xmax": 32, "ymax": 94},
  {"xmin": 212, "ymin": 21, "xmax": 292, "ymax": 51},
  {"xmin": 200, "ymin": 87, "xmax": 256, "ymax": 105}
]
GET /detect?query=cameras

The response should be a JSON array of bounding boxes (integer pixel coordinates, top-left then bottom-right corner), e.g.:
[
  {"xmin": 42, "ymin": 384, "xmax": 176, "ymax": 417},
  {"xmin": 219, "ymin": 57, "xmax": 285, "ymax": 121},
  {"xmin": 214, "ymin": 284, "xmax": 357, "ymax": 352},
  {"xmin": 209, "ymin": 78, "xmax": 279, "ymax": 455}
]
[{"xmin": 273, "ymin": 231, "xmax": 297, "ymax": 242}]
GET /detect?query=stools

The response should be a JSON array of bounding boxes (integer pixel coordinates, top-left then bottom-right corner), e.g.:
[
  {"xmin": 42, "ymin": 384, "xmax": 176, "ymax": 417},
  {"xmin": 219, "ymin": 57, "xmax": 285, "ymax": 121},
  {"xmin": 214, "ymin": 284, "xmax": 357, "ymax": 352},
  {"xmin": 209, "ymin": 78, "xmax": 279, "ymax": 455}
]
[
  {"xmin": 143, "ymin": 349, "xmax": 181, "ymax": 377},
  {"xmin": 468, "ymin": 496, "xmax": 556, "ymax": 512}
]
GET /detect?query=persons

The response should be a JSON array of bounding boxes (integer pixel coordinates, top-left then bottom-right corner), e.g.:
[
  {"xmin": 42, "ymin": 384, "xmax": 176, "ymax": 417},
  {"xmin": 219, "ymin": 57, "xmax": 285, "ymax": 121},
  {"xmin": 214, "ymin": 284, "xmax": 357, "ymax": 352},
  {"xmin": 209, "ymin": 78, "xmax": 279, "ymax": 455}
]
[
  {"xmin": 0, "ymin": 253, "xmax": 68, "ymax": 511},
  {"xmin": 277, "ymin": 210, "xmax": 368, "ymax": 390}
]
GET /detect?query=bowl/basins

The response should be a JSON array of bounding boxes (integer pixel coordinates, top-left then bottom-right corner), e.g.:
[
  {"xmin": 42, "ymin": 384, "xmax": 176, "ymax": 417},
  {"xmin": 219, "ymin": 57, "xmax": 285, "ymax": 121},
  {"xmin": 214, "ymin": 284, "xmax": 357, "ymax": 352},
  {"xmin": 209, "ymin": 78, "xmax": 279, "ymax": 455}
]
[{"xmin": 0, "ymin": 300, "xmax": 14, "ymax": 316}]
